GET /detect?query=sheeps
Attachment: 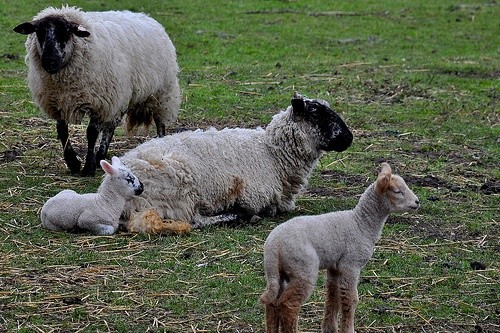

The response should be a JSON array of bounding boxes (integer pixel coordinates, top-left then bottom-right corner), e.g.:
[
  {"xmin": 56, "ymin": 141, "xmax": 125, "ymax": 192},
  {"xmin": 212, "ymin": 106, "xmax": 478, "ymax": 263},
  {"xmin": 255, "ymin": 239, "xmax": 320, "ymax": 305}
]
[
  {"xmin": 119, "ymin": 91, "xmax": 353, "ymax": 235},
  {"xmin": 41, "ymin": 156, "xmax": 144, "ymax": 235},
  {"xmin": 12, "ymin": 3, "xmax": 183, "ymax": 178},
  {"xmin": 257, "ymin": 161, "xmax": 420, "ymax": 333}
]
